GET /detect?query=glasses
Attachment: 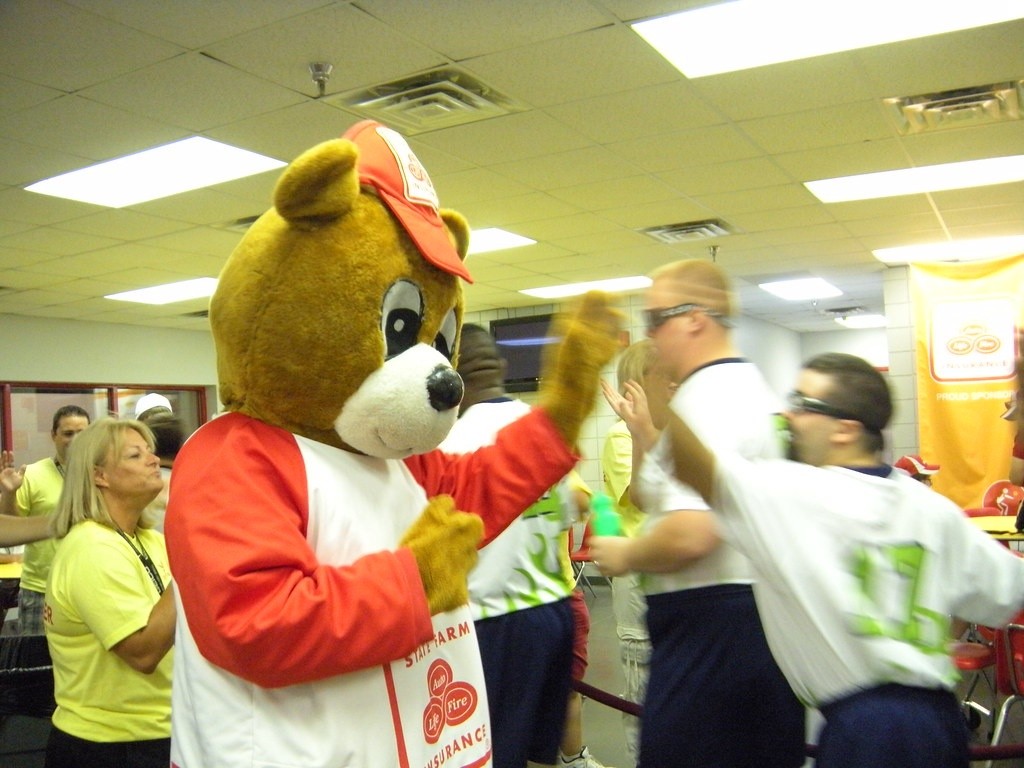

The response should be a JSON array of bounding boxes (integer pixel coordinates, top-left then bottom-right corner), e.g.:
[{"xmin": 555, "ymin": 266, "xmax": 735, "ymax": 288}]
[
  {"xmin": 788, "ymin": 393, "xmax": 875, "ymax": 432},
  {"xmin": 642, "ymin": 302, "xmax": 737, "ymax": 329}
]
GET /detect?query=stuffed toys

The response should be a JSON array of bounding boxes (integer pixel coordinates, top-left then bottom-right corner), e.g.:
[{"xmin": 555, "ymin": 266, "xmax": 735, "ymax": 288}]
[{"xmin": 162, "ymin": 119, "xmax": 631, "ymax": 767}]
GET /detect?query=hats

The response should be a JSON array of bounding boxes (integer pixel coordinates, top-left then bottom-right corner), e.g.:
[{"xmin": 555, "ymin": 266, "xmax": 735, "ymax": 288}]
[
  {"xmin": 894, "ymin": 455, "xmax": 940, "ymax": 477},
  {"xmin": 135, "ymin": 393, "xmax": 172, "ymax": 420}
]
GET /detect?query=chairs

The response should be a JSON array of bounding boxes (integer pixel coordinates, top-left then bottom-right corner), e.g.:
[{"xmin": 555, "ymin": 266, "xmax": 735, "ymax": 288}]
[
  {"xmin": 986, "ymin": 610, "xmax": 1024, "ymax": 768},
  {"xmin": 964, "ymin": 508, "xmax": 1021, "ymax": 558},
  {"xmin": 954, "ymin": 623, "xmax": 1017, "ymax": 744}
]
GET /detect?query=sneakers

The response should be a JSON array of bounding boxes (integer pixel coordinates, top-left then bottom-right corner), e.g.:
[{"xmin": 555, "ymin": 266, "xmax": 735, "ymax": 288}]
[{"xmin": 559, "ymin": 745, "xmax": 615, "ymax": 768}]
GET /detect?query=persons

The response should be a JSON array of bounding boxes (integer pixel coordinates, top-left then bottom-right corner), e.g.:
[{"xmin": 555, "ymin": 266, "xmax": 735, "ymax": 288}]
[
  {"xmin": 437, "ymin": 325, "xmax": 572, "ymax": 767},
  {"xmin": 0, "ymin": 406, "xmax": 190, "ymax": 634},
  {"xmin": 584, "ymin": 262, "xmax": 805, "ymax": 768},
  {"xmin": 134, "ymin": 393, "xmax": 174, "ymax": 421},
  {"xmin": 559, "ymin": 261, "xmax": 1024, "ymax": 768},
  {"xmin": 42, "ymin": 416, "xmax": 177, "ymax": 768}
]
